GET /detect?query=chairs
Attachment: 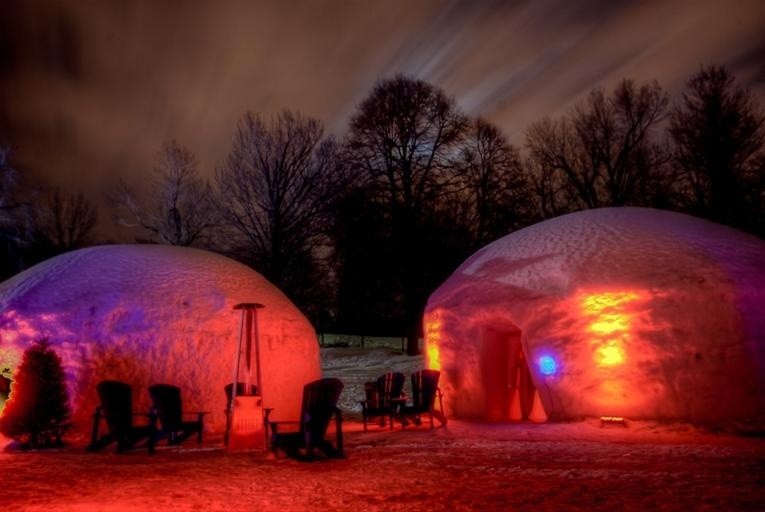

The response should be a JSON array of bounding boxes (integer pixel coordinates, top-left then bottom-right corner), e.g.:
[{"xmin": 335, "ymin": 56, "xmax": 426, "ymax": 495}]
[
  {"xmin": 268, "ymin": 378, "xmax": 344, "ymax": 463},
  {"xmin": 85, "ymin": 380, "xmax": 156, "ymax": 454},
  {"xmin": 398, "ymin": 369, "xmax": 446, "ymax": 430},
  {"xmin": 360, "ymin": 382, "xmax": 410, "ymax": 432},
  {"xmin": 147, "ymin": 384, "xmax": 209, "ymax": 453},
  {"xmin": 228, "ymin": 396, "xmax": 268, "ymax": 454},
  {"xmin": 369, "ymin": 371, "xmax": 405, "ymax": 429}
]
